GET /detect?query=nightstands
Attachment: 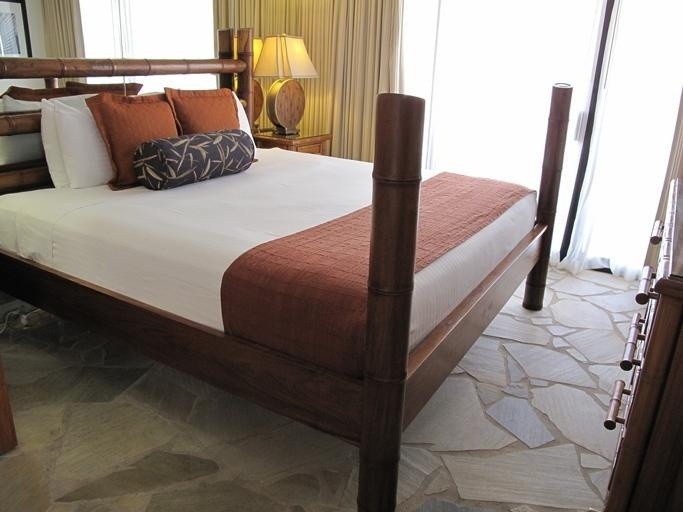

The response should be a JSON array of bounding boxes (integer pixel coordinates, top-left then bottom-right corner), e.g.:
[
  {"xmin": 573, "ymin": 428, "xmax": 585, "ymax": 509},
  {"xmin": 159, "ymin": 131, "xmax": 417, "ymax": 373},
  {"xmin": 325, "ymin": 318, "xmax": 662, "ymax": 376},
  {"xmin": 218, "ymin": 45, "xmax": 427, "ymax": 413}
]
[{"xmin": 254, "ymin": 131, "xmax": 330, "ymax": 158}]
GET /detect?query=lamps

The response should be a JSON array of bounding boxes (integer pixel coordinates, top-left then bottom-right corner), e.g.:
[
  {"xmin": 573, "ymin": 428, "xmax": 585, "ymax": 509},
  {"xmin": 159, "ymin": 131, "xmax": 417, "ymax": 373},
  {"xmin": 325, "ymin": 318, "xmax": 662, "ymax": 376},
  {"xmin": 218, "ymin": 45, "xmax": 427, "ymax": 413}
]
[{"xmin": 253, "ymin": 33, "xmax": 321, "ymax": 135}]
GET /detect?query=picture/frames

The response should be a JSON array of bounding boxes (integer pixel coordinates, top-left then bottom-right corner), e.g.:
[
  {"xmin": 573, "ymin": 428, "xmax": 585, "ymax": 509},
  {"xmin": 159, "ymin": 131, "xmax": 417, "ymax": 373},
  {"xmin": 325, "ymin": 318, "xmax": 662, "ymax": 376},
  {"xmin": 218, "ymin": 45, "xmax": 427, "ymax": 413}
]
[{"xmin": 0, "ymin": 0, "xmax": 33, "ymax": 59}]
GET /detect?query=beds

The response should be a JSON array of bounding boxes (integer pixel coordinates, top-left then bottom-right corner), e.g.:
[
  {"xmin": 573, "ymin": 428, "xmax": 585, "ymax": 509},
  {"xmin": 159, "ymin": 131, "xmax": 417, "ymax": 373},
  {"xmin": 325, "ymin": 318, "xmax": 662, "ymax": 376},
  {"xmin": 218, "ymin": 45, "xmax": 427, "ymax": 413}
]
[{"xmin": 2, "ymin": 27, "xmax": 575, "ymax": 512}]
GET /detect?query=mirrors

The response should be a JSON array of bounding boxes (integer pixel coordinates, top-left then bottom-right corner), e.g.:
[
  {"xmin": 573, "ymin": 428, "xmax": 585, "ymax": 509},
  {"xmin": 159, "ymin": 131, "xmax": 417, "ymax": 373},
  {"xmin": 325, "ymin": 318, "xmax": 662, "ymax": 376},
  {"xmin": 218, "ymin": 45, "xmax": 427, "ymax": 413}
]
[{"xmin": 0, "ymin": 0, "xmax": 240, "ymax": 169}]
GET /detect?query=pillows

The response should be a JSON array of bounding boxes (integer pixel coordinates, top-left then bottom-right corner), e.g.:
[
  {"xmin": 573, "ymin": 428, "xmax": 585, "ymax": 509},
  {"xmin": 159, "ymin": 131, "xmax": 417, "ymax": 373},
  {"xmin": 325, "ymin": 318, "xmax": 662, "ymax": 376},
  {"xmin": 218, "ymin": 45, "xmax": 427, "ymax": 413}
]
[
  {"xmin": 41, "ymin": 83, "xmax": 257, "ymax": 191},
  {"xmin": 0, "ymin": 81, "xmax": 72, "ymax": 168}
]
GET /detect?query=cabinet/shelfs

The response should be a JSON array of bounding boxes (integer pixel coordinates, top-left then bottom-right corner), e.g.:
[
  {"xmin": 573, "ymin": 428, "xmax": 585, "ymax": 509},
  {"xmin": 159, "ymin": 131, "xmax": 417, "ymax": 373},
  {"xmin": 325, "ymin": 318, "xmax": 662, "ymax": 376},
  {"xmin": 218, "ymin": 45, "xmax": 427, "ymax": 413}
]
[{"xmin": 600, "ymin": 177, "xmax": 681, "ymax": 511}]
